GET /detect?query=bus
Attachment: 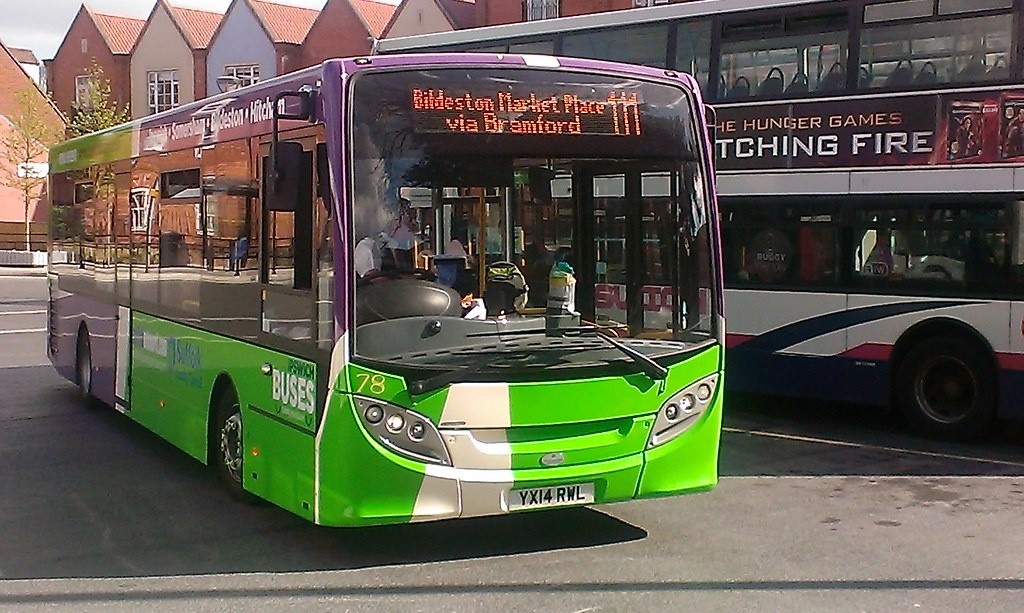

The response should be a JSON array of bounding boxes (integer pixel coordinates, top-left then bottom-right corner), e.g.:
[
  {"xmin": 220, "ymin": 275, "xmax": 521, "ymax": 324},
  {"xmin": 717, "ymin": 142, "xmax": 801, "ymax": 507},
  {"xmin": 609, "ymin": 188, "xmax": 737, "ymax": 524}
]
[
  {"xmin": 365, "ymin": 0, "xmax": 1023, "ymax": 444},
  {"xmin": 47, "ymin": 53, "xmax": 726, "ymax": 528}
]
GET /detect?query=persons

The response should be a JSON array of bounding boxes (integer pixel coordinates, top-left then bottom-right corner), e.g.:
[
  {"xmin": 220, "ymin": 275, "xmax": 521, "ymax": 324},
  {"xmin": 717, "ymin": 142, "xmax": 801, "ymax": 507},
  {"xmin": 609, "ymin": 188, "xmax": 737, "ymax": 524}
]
[
  {"xmin": 355, "ymin": 196, "xmax": 412, "ymax": 278},
  {"xmin": 869, "ymin": 211, "xmax": 995, "ymax": 280},
  {"xmin": 959, "ymin": 105, "xmax": 1024, "ymax": 156}
]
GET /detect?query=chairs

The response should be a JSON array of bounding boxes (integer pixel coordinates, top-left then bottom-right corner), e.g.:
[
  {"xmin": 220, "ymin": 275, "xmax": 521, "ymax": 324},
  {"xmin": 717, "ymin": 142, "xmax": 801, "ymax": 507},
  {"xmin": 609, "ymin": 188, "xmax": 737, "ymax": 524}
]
[
  {"xmin": 436, "ymin": 256, "xmax": 528, "ymax": 308},
  {"xmin": 703, "ymin": 53, "xmax": 1009, "ymax": 104}
]
